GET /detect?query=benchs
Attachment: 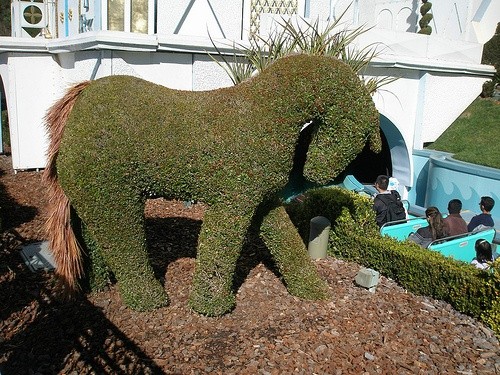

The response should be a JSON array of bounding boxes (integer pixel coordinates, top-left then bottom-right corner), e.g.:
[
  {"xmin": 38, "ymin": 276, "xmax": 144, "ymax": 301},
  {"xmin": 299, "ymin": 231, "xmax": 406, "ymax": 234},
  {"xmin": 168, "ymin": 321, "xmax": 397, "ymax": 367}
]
[
  {"xmin": 426, "ymin": 229, "xmax": 496, "ymax": 264},
  {"xmin": 378, "ymin": 213, "xmax": 446, "ymax": 243}
]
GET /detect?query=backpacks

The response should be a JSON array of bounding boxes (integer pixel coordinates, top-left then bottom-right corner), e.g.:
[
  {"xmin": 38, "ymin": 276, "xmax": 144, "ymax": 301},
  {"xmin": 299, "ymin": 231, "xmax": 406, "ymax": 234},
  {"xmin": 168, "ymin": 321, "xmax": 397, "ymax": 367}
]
[{"xmin": 376, "ymin": 191, "xmax": 406, "ymax": 226}]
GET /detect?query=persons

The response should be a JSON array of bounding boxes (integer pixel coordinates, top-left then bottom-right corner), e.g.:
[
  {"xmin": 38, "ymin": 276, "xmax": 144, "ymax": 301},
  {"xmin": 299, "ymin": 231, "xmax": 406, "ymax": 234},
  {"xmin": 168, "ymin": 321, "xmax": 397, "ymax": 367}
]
[
  {"xmin": 408, "ymin": 206, "xmax": 445, "ymax": 248},
  {"xmin": 373, "ymin": 176, "xmax": 401, "ymax": 227},
  {"xmin": 471, "ymin": 239, "xmax": 494, "ymax": 271},
  {"xmin": 467, "ymin": 196, "xmax": 495, "ymax": 232},
  {"xmin": 443, "ymin": 199, "xmax": 468, "ymax": 236}
]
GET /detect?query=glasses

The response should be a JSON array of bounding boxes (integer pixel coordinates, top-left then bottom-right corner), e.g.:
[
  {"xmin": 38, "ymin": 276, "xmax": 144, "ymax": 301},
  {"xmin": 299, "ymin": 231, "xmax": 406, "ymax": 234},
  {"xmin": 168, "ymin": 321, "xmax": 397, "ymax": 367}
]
[{"xmin": 479, "ymin": 203, "xmax": 481, "ymax": 205}]
